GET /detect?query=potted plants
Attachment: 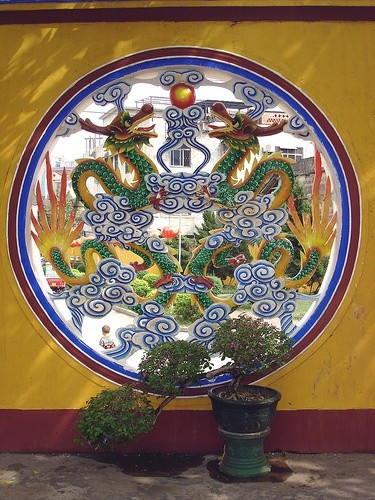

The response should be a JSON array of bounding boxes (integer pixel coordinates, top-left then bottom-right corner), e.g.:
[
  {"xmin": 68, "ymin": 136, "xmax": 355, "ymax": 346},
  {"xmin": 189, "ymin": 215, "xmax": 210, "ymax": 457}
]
[{"xmin": 72, "ymin": 314, "xmax": 297, "ymax": 481}]
